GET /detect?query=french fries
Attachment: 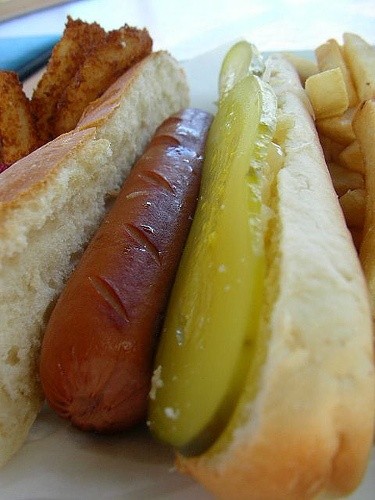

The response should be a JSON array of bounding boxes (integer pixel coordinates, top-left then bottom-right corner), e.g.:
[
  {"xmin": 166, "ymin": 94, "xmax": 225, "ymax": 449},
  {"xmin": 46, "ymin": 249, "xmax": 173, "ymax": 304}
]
[{"xmin": 292, "ymin": 34, "xmax": 375, "ymax": 315}]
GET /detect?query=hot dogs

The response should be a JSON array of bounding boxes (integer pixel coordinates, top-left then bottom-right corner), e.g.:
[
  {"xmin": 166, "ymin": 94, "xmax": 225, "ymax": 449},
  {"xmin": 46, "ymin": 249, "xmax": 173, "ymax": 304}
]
[{"xmin": 0, "ymin": 56, "xmax": 375, "ymax": 496}]
